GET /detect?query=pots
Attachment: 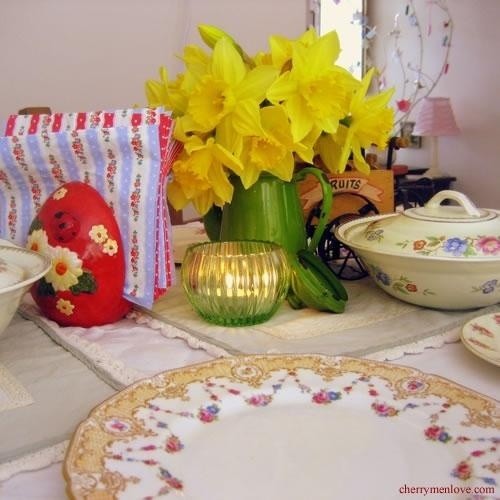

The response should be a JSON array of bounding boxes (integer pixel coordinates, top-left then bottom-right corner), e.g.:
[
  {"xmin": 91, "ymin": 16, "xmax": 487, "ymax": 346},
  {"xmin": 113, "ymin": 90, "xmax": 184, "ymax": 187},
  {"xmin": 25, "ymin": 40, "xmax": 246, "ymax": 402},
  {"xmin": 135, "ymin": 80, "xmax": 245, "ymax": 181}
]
[{"xmin": 335, "ymin": 188, "xmax": 500, "ymax": 313}]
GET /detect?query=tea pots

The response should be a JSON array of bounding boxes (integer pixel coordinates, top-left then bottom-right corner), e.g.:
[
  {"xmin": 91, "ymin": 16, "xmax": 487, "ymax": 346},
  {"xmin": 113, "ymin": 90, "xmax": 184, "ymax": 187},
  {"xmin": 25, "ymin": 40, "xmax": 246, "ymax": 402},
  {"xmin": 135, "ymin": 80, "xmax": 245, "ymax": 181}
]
[{"xmin": 202, "ymin": 167, "xmax": 331, "ymax": 308}]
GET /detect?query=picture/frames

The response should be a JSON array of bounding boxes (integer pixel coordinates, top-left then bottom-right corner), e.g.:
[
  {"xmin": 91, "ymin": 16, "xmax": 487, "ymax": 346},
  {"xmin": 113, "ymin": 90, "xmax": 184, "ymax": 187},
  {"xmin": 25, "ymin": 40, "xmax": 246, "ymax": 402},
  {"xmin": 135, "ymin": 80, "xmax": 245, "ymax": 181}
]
[{"xmin": 400, "ymin": 121, "xmax": 422, "ymax": 148}]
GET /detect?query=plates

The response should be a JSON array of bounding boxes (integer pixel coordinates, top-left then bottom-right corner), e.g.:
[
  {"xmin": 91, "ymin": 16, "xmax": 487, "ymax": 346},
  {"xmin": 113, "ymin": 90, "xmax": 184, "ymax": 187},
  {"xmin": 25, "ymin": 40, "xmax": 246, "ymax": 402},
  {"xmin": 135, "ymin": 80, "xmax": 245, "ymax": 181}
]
[
  {"xmin": 63, "ymin": 351, "xmax": 498, "ymax": 499},
  {"xmin": 461, "ymin": 311, "xmax": 500, "ymax": 365}
]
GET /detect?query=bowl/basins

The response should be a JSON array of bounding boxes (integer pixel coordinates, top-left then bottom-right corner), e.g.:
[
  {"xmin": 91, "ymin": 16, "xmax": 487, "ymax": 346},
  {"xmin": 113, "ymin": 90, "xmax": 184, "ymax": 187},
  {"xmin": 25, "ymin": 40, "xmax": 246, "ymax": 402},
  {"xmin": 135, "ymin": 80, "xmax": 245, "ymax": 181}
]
[
  {"xmin": 0, "ymin": 243, "xmax": 52, "ymax": 335},
  {"xmin": 180, "ymin": 240, "xmax": 293, "ymax": 327}
]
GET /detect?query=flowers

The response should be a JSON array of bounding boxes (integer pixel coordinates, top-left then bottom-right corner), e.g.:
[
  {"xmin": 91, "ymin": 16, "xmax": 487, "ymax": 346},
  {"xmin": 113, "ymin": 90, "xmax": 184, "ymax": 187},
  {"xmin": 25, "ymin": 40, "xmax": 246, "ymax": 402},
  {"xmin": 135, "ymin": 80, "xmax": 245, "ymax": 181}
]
[{"xmin": 143, "ymin": 17, "xmax": 404, "ymax": 217}]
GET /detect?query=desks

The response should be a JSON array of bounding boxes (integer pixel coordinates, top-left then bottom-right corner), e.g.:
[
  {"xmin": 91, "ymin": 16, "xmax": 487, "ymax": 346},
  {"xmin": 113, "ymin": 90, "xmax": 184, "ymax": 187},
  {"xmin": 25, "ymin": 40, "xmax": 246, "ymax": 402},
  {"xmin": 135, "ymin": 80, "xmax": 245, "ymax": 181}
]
[{"xmin": 395, "ymin": 173, "xmax": 458, "ymax": 206}]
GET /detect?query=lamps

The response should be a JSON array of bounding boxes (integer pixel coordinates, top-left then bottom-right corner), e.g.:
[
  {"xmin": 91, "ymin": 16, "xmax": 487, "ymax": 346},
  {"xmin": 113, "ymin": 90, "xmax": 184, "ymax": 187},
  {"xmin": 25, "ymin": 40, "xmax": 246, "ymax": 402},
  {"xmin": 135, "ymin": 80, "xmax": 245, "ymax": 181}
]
[{"xmin": 411, "ymin": 98, "xmax": 461, "ymax": 178}]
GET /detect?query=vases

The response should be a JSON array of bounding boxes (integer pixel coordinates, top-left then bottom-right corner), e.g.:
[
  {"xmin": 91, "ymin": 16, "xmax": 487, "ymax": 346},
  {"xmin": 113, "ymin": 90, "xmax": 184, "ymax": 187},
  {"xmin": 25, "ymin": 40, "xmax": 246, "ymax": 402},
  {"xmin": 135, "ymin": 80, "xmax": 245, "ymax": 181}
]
[{"xmin": 202, "ymin": 163, "xmax": 350, "ymax": 314}]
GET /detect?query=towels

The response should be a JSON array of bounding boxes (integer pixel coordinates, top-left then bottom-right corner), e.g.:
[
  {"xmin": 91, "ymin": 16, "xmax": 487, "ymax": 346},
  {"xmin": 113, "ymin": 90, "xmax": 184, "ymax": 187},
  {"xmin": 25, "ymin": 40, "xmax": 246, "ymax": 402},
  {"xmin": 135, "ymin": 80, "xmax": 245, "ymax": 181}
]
[{"xmin": 0, "ymin": 105, "xmax": 184, "ymax": 308}]
